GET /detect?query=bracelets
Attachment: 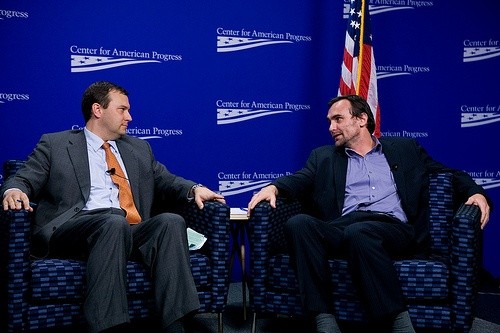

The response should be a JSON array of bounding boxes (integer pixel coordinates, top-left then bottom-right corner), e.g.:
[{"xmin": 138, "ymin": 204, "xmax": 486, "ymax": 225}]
[{"xmin": 192, "ymin": 183, "xmax": 203, "ymax": 195}]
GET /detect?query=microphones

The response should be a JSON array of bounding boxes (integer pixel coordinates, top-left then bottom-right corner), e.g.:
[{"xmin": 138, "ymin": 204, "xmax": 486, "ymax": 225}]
[
  {"xmin": 391, "ymin": 163, "xmax": 398, "ymax": 171},
  {"xmin": 105, "ymin": 168, "xmax": 116, "ymax": 174}
]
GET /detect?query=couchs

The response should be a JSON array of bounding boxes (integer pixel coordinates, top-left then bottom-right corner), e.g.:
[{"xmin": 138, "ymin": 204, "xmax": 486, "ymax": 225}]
[
  {"xmin": 4, "ymin": 160, "xmax": 229, "ymax": 333},
  {"xmin": 249, "ymin": 174, "xmax": 483, "ymax": 333}
]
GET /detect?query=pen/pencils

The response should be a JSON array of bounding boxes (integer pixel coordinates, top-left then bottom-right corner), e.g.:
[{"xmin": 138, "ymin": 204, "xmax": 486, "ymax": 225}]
[
  {"xmin": 240, "ymin": 208, "xmax": 248, "ymax": 211},
  {"xmin": 17, "ymin": 200, "xmax": 40, "ymax": 207}
]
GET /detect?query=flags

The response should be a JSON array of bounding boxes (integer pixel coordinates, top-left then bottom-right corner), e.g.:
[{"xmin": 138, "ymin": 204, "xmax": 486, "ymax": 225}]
[{"xmin": 337, "ymin": 0, "xmax": 380, "ymax": 139}]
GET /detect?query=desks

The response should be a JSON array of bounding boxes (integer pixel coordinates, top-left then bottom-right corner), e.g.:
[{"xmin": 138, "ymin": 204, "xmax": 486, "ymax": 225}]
[{"xmin": 228, "ymin": 207, "xmax": 248, "ymax": 320}]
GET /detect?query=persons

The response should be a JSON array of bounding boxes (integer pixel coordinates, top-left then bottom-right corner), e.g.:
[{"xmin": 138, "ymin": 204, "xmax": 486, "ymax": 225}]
[
  {"xmin": 248, "ymin": 95, "xmax": 490, "ymax": 333},
  {"xmin": 0, "ymin": 81, "xmax": 226, "ymax": 333}
]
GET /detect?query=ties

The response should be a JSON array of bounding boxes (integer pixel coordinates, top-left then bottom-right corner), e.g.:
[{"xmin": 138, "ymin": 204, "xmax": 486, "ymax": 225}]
[{"xmin": 102, "ymin": 143, "xmax": 141, "ymax": 224}]
